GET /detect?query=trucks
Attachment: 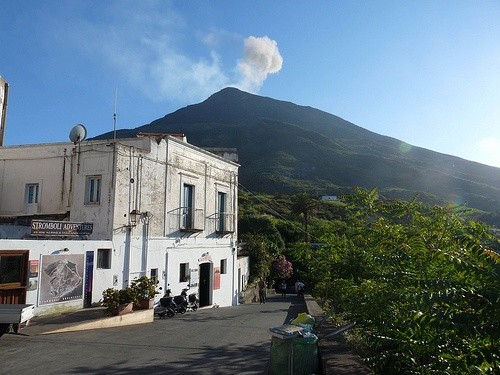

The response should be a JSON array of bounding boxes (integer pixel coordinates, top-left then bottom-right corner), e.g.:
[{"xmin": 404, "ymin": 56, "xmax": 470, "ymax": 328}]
[{"xmin": 0, "ymin": 303, "xmax": 36, "ymax": 337}]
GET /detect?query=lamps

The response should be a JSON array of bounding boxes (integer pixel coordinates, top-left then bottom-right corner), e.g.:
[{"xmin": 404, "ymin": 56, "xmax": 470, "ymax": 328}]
[{"xmin": 123, "ymin": 208, "xmax": 142, "ymax": 231}]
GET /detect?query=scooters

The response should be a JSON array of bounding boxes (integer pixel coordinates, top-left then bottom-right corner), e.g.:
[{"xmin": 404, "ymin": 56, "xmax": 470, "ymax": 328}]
[{"xmin": 154, "ymin": 283, "xmax": 200, "ymax": 319}]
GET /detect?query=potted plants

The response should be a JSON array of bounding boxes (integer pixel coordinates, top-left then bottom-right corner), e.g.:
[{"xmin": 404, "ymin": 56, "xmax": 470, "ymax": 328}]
[{"xmin": 102, "ymin": 275, "xmax": 161, "ymax": 317}]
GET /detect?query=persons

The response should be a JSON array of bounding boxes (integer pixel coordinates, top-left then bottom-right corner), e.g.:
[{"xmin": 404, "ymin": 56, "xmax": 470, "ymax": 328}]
[
  {"xmin": 280, "ymin": 280, "xmax": 288, "ymax": 299},
  {"xmin": 258, "ymin": 277, "xmax": 268, "ymax": 304},
  {"xmin": 294, "ymin": 279, "xmax": 304, "ymax": 295}
]
